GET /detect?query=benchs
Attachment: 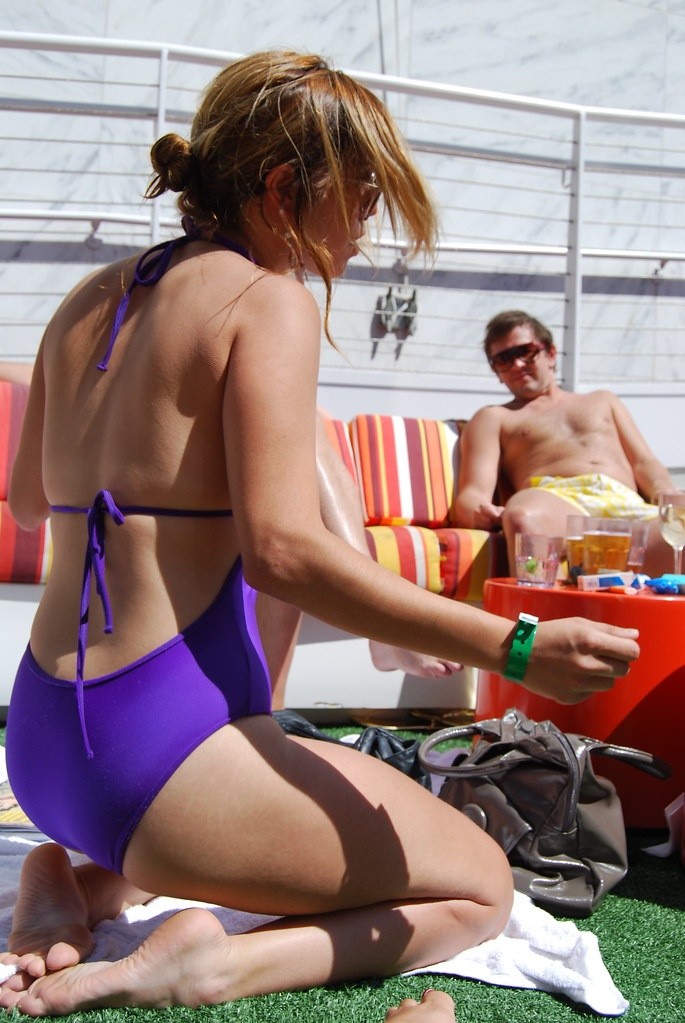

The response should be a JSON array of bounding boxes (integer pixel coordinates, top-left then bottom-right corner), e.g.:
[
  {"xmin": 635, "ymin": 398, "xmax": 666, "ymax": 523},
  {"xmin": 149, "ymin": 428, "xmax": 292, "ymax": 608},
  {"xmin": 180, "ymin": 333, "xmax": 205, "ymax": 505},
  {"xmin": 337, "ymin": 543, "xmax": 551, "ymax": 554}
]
[
  {"xmin": 0, "ymin": 378, "xmax": 54, "ymax": 584},
  {"xmin": 316, "ymin": 415, "xmax": 508, "ymax": 604}
]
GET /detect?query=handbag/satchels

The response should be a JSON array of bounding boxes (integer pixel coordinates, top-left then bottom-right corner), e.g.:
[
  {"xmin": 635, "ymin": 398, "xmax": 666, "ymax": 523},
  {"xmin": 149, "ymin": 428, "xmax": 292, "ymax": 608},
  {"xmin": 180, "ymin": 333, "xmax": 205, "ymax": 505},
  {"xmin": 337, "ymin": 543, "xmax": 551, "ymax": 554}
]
[{"xmin": 416, "ymin": 706, "xmax": 673, "ymax": 918}]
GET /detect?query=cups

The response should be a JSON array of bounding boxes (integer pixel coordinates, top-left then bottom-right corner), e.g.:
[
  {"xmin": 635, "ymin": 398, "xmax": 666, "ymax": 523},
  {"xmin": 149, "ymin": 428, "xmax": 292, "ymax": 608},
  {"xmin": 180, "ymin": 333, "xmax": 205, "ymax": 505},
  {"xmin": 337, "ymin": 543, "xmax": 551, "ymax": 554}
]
[
  {"xmin": 625, "ymin": 520, "xmax": 649, "ymax": 574},
  {"xmin": 544, "ymin": 537, "xmax": 564, "ymax": 587},
  {"xmin": 563, "ymin": 514, "xmax": 586, "ymax": 586},
  {"xmin": 515, "ymin": 534, "xmax": 548, "ymax": 588},
  {"xmin": 583, "ymin": 518, "xmax": 632, "ymax": 574}
]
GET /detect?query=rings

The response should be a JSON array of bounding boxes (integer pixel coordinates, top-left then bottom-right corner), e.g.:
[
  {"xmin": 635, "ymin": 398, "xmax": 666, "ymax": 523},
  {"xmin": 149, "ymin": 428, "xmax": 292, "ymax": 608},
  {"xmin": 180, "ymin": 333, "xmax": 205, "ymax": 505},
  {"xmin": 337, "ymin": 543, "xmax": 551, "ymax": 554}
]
[{"xmin": 422, "ymin": 989, "xmax": 433, "ymax": 998}]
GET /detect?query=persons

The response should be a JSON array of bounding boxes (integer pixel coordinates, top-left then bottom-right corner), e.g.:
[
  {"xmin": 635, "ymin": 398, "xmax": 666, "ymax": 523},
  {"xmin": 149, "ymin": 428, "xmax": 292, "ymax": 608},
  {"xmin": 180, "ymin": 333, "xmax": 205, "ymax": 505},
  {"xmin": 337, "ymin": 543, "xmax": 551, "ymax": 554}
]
[
  {"xmin": 451, "ymin": 311, "xmax": 685, "ymax": 580},
  {"xmin": 255, "ymin": 412, "xmax": 464, "ymax": 712},
  {"xmin": 386, "ymin": 988, "xmax": 456, "ymax": 1023},
  {"xmin": 0, "ymin": 52, "xmax": 640, "ymax": 1016}
]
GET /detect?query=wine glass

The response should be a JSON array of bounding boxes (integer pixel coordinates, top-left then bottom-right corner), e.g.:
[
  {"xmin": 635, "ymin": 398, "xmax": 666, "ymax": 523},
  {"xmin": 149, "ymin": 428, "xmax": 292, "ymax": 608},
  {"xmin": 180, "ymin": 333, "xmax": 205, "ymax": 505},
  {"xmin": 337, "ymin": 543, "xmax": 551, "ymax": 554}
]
[{"xmin": 655, "ymin": 483, "xmax": 685, "ymax": 574}]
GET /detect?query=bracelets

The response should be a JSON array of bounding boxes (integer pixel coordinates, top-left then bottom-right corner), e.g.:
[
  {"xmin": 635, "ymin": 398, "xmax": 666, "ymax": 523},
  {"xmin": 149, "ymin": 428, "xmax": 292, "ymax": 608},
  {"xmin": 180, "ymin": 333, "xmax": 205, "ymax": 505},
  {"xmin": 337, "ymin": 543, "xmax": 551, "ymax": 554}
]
[{"xmin": 502, "ymin": 612, "xmax": 539, "ymax": 684}]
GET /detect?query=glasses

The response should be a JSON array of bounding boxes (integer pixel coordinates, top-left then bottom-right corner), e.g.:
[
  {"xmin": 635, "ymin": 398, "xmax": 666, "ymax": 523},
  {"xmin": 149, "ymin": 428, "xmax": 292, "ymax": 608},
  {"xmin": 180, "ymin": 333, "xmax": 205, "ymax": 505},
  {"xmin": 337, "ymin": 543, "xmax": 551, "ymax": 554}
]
[
  {"xmin": 490, "ymin": 343, "xmax": 546, "ymax": 373},
  {"xmin": 295, "ymin": 166, "xmax": 382, "ymax": 221}
]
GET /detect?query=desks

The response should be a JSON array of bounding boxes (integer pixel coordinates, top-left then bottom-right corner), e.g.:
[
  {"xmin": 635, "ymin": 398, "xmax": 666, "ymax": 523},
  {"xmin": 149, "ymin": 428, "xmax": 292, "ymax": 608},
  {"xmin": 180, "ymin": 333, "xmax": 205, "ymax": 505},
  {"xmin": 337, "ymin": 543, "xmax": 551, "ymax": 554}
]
[{"xmin": 474, "ymin": 576, "xmax": 685, "ymax": 828}]
